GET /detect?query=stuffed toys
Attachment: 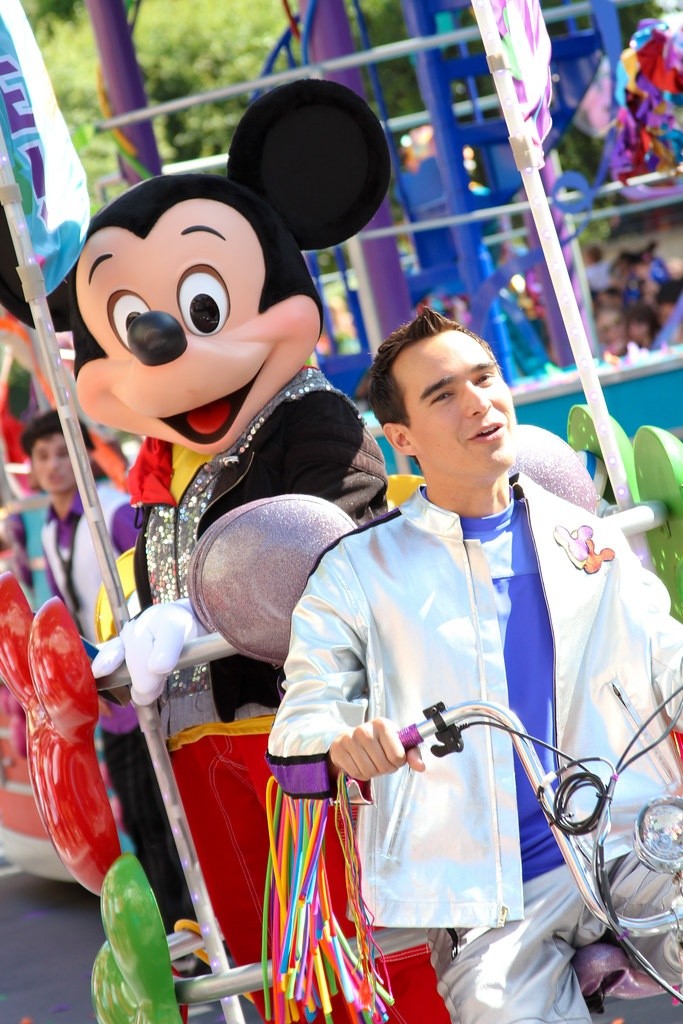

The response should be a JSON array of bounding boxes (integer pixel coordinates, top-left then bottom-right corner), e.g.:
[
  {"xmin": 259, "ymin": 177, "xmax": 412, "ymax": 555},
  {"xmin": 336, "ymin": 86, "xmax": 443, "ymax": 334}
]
[{"xmin": 0, "ymin": 78, "xmax": 450, "ymax": 1024}]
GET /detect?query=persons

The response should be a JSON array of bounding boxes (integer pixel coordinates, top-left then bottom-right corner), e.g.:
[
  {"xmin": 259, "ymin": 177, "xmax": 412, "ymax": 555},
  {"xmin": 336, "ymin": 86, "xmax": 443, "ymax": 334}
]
[
  {"xmin": 18, "ymin": 407, "xmax": 218, "ymax": 1018},
  {"xmin": 267, "ymin": 307, "xmax": 683, "ymax": 1023},
  {"xmin": 579, "ymin": 242, "xmax": 683, "ymax": 358}
]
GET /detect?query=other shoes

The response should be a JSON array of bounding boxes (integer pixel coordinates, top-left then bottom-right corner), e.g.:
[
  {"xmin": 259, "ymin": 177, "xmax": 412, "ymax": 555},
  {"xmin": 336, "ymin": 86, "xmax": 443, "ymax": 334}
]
[{"xmin": 168, "ymin": 957, "xmax": 213, "ymax": 1018}]
